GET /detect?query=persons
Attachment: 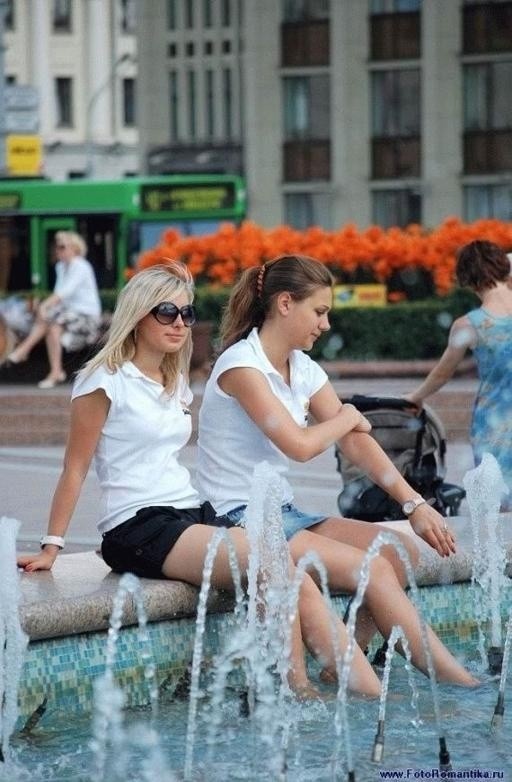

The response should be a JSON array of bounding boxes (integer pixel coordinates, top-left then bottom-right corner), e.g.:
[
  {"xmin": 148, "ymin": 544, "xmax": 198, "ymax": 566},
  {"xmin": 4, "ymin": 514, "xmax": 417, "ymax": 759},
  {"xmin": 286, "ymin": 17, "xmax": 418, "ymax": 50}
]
[
  {"xmin": 15, "ymin": 260, "xmax": 388, "ymax": 698},
  {"xmin": 401, "ymin": 240, "xmax": 511, "ymax": 515},
  {"xmin": 5, "ymin": 231, "xmax": 104, "ymax": 388},
  {"xmin": 193, "ymin": 254, "xmax": 481, "ymax": 691}
]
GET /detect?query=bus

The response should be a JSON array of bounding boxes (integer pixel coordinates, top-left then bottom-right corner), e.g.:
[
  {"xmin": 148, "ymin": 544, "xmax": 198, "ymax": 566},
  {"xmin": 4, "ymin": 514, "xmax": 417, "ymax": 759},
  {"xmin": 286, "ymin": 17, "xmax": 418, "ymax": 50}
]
[{"xmin": 0, "ymin": 173, "xmax": 245, "ymax": 315}]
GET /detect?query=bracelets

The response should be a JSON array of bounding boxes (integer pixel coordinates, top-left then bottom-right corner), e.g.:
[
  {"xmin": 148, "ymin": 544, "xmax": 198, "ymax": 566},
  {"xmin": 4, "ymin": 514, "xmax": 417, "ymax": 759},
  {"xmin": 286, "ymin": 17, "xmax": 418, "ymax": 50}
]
[{"xmin": 38, "ymin": 534, "xmax": 64, "ymax": 550}]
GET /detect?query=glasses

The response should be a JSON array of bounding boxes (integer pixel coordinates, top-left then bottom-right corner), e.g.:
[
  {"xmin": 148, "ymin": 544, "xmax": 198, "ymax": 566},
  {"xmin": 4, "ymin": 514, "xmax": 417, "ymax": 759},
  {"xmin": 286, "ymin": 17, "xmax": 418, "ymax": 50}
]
[{"xmin": 150, "ymin": 301, "xmax": 197, "ymax": 327}]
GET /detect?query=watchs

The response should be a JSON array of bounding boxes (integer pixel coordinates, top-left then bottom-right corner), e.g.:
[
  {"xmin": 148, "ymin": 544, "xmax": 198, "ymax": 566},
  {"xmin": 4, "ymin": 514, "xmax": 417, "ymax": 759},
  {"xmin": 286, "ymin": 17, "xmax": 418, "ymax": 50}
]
[{"xmin": 402, "ymin": 495, "xmax": 424, "ymax": 514}]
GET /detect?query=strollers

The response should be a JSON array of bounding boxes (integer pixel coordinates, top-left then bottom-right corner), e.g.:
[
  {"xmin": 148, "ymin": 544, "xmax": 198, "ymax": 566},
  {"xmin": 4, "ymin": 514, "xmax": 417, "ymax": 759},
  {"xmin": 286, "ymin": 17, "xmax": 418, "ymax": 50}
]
[{"xmin": 334, "ymin": 395, "xmax": 466, "ymax": 523}]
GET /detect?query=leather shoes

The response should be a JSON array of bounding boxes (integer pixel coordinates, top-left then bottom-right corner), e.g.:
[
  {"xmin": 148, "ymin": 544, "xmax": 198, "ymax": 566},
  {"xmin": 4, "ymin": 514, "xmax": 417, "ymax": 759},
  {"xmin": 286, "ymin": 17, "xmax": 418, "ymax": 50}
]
[
  {"xmin": 37, "ymin": 369, "xmax": 67, "ymax": 390},
  {"xmin": 8, "ymin": 347, "xmax": 28, "ymax": 364}
]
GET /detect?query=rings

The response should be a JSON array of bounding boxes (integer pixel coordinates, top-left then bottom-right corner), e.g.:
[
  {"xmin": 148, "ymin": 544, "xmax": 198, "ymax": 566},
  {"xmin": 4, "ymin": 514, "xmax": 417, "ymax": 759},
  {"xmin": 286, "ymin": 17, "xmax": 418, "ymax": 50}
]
[{"xmin": 441, "ymin": 525, "xmax": 449, "ymax": 531}]
